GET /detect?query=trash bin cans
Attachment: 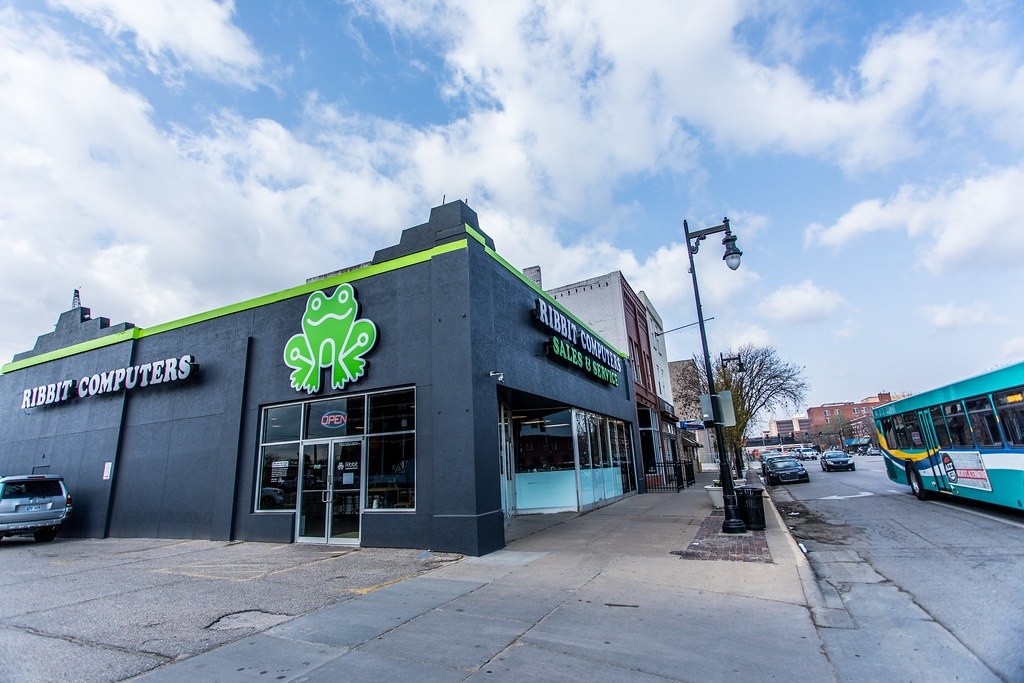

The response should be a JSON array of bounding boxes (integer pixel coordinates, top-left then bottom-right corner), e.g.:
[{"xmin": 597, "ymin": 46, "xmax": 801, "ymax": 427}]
[{"xmin": 733, "ymin": 485, "xmax": 767, "ymax": 531}]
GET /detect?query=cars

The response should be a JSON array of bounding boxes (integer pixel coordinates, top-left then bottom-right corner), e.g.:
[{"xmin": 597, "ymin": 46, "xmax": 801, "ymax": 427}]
[
  {"xmin": 820, "ymin": 451, "xmax": 855, "ymax": 472},
  {"xmin": 799, "ymin": 448, "xmax": 817, "ymax": 461},
  {"xmin": 262, "ymin": 486, "xmax": 284, "ymax": 509},
  {"xmin": 789, "ymin": 448, "xmax": 806, "ymax": 459},
  {"xmin": 767, "ymin": 460, "xmax": 809, "ymax": 486},
  {"xmin": 759, "ymin": 453, "xmax": 793, "ymax": 477}
]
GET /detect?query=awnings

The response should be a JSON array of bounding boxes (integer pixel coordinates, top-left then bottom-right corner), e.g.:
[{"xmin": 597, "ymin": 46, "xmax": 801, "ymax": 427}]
[
  {"xmin": 691, "ymin": 439, "xmax": 704, "ymax": 448},
  {"xmin": 682, "ymin": 437, "xmax": 696, "ymax": 446}
]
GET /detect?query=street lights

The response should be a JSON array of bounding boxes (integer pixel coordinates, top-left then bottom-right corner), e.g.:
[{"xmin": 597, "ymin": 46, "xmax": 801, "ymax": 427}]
[{"xmin": 683, "ymin": 219, "xmax": 747, "ymax": 533}]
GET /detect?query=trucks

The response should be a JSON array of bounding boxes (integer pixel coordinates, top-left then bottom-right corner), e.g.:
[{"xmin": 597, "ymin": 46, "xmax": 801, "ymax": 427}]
[{"xmin": 867, "ymin": 447, "xmax": 880, "ymax": 456}]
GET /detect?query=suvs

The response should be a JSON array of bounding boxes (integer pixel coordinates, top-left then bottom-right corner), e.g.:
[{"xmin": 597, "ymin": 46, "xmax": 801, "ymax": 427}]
[
  {"xmin": 859, "ymin": 445, "xmax": 871, "ymax": 456},
  {"xmin": 0, "ymin": 475, "xmax": 73, "ymax": 542}
]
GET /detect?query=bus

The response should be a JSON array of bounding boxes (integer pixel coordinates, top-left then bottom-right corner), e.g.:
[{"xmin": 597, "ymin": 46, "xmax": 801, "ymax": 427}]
[
  {"xmin": 766, "ymin": 444, "xmax": 803, "ymax": 456},
  {"xmin": 871, "ymin": 361, "xmax": 1024, "ymax": 510}
]
